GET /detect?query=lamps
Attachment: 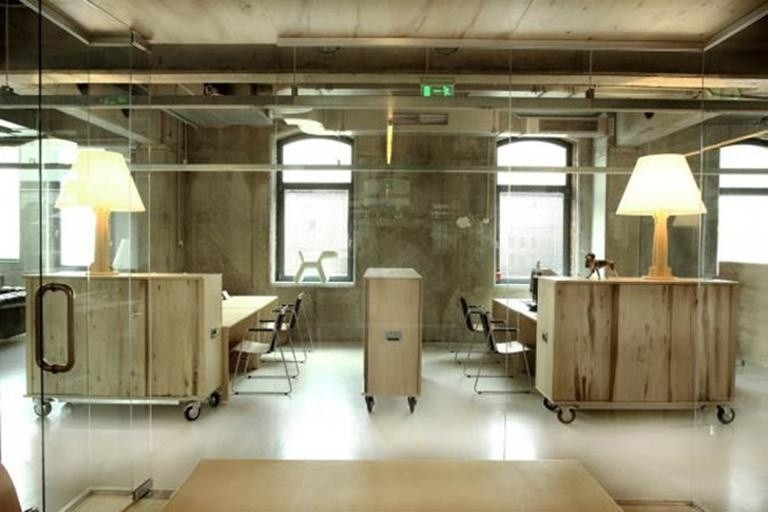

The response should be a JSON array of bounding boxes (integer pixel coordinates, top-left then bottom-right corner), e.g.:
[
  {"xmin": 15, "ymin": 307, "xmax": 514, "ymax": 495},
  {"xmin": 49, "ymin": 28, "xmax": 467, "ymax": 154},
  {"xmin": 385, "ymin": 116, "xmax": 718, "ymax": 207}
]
[
  {"xmin": 616, "ymin": 153, "xmax": 708, "ymax": 282},
  {"xmin": 54, "ymin": 149, "xmax": 147, "ymax": 275}
]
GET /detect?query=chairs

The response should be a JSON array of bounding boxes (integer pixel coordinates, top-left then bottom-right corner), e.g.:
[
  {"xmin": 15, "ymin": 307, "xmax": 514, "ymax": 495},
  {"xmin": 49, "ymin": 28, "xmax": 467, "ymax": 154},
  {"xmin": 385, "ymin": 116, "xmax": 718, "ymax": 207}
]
[
  {"xmin": 455, "ymin": 296, "xmax": 534, "ymax": 394},
  {"xmin": 230, "ymin": 292, "xmax": 313, "ymax": 395}
]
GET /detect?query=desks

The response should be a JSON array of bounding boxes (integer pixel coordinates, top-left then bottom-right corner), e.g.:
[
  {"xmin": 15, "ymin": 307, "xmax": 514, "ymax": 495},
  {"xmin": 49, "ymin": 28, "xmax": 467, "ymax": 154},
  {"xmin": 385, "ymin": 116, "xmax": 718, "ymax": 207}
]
[
  {"xmin": 535, "ymin": 276, "xmax": 741, "ymax": 425},
  {"xmin": 221, "ymin": 295, "xmax": 280, "ymax": 398},
  {"xmin": 22, "ymin": 273, "xmax": 223, "ymax": 421},
  {"xmin": 161, "ymin": 457, "xmax": 624, "ymax": 512}
]
